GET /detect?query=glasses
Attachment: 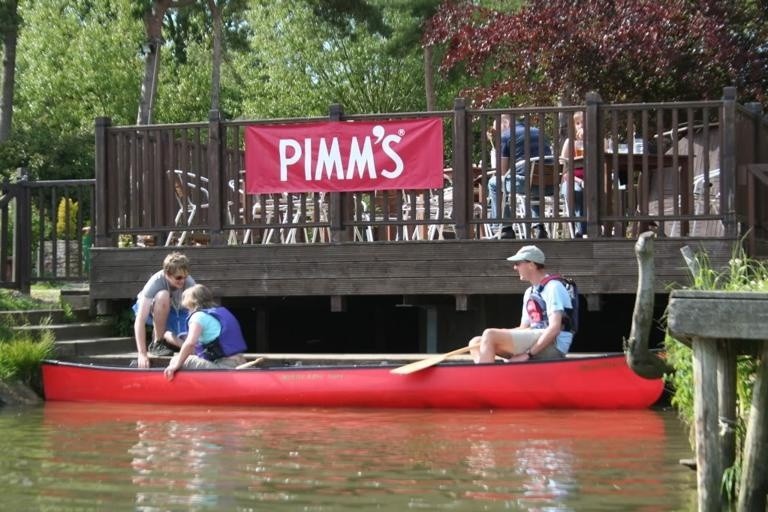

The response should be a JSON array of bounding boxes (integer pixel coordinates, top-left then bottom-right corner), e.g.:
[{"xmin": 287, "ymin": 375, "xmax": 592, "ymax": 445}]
[
  {"xmin": 515, "ymin": 261, "xmax": 528, "ymax": 265},
  {"xmin": 170, "ymin": 274, "xmax": 188, "ymax": 280}
]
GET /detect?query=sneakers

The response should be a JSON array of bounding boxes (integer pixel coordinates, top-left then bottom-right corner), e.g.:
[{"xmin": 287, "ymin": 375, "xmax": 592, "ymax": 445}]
[
  {"xmin": 148, "ymin": 341, "xmax": 174, "ymax": 356},
  {"xmin": 493, "ymin": 229, "xmax": 516, "ymax": 239},
  {"xmin": 536, "ymin": 228, "xmax": 548, "ymax": 237}
]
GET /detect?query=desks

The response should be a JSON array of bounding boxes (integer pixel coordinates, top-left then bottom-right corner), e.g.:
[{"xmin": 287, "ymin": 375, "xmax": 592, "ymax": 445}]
[{"xmin": 573, "ymin": 153, "xmax": 698, "ymax": 239}]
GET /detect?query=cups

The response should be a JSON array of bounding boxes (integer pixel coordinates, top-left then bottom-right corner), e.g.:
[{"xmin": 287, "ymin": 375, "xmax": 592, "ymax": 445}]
[{"xmin": 573, "ymin": 139, "xmax": 583, "ymax": 158}]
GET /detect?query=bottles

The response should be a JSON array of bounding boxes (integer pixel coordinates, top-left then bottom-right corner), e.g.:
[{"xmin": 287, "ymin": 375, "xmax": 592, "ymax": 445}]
[{"xmin": 603, "ymin": 135, "xmax": 613, "ymax": 155}]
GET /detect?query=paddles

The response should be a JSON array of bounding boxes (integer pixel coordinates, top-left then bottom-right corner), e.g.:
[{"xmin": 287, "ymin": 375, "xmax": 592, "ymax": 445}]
[{"xmin": 391, "ymin": 343, "xmax": 480, "ymax": 375}]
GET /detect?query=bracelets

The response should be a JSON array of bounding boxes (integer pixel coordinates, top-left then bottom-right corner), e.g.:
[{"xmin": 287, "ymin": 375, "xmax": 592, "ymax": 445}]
[{"xmin": 526, "ymin": 348, "xmax": 534, "ymax": 360}]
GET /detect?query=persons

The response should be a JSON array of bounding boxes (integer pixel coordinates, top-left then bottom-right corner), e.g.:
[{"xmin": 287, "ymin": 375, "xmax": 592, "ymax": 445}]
[
  {"xmin": 162, "ymin": 283, "xmax": 248, "ymax": 382},
  {"xmin": 466, "ymin": 243, "xmax": 581, "ymax": 365},
  {"xmin": 131, "ymin": 250, "xmax": 199, "ymax": 369},
  {"xmin": 488, "ymin": 111, "xmax": 553, "ymax": 240},
  {"xmin": 556, "ymin": 110, "xmax": 588, "ymax": 239}
]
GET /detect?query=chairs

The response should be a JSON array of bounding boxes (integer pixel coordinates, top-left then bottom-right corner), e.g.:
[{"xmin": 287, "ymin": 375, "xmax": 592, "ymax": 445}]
[
  {"xmin": 163, "ymin": 154, "xmax": 578, "ymax": 246},
  {"xmin": 639, "ymin": 168, "xmax": 726, "ymax": 237}
]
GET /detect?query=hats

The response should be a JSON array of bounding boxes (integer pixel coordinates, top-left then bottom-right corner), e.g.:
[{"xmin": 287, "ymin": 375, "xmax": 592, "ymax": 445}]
[{"xmin": 507, "ymin": 245, "xmax": 545, "ymax": 265}]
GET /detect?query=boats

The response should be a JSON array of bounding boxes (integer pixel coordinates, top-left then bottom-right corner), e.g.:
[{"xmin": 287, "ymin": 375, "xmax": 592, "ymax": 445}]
[{"xmin": 37, "ymin": 349, "xmax": 669, "ymax": 408}]
